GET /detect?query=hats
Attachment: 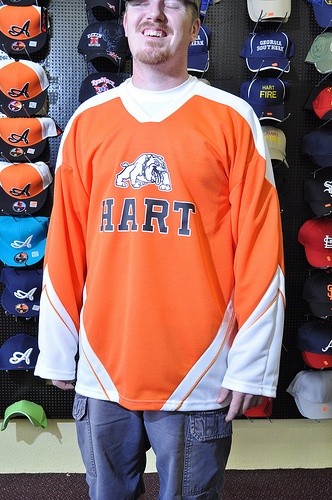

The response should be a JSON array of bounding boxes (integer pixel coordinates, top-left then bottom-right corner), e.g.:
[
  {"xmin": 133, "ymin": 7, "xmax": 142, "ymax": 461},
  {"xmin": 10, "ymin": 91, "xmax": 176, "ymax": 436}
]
[
  {"xmin": 78, "ymin": 0, "xmax": 124, "ymax": 104},
  {"xmin": 187, "ymin": 0, "xmax": 212, "ymax": 74},
  {"xmin": 285, "ymin": 0, "xmax": 332, "ymax": 419},
  {"xmin": 0, "ymin": 1, "xmax": 63, "ymax": 429},
  {"xmin": 240, "ymin": 0, "xmax": 291, "ymax": 169}
]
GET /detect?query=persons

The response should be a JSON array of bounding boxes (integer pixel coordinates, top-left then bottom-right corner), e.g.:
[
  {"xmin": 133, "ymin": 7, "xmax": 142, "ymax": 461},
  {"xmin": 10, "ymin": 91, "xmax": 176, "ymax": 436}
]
[{"xmin": 34, "ymin": 0, "xmax": 286, "ymax": 500}]
